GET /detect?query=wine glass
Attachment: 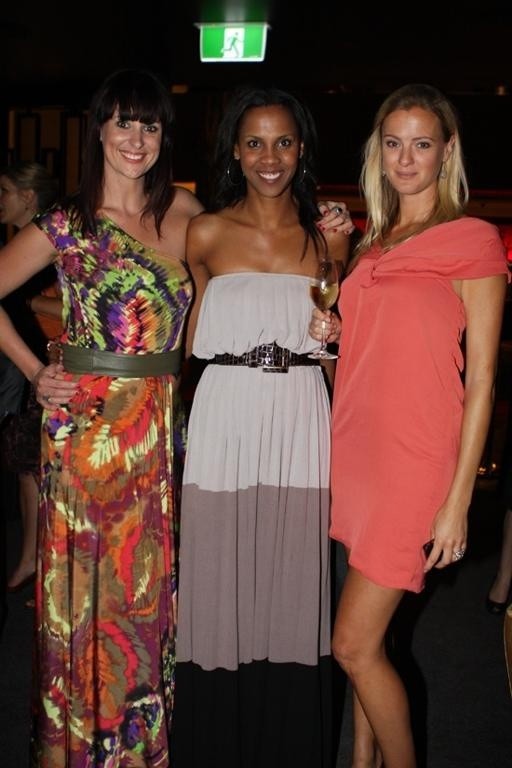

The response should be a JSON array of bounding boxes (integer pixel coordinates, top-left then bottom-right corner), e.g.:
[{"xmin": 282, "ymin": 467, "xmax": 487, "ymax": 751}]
[{"xmin": 307, "ymin": 257, "xmax": 340, "ymax": 359}]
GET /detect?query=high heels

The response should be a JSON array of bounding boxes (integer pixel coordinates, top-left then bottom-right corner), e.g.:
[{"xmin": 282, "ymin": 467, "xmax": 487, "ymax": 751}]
[{"xmin": 3, "ymin": 570, "xmax": 37, "ymax": 596}]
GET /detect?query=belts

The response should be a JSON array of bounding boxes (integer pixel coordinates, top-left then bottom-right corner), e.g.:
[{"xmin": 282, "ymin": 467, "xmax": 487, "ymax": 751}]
[{"xmin": 209, "ymin": 345, "xmax": 321, "ymax": 372}]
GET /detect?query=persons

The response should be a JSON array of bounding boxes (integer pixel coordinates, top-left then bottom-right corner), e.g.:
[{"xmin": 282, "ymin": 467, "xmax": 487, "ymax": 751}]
[
  {"xmin": 328, "ymin": 82, "xmax": 511, "ymax": 768},
  {"xmin": 0, "ymin": 66, "xmax": 356, "ymax": 767},
  {"xmin": 484, "ymin": 484, "xmax": 511, "ymax": 615},
  {"xmin": 0, "ymin": 160, "xmax": 58, "ymax": 614},
  {"xmin": 187, "ymin": 78, "xmax": 350, "ymax": 765}
]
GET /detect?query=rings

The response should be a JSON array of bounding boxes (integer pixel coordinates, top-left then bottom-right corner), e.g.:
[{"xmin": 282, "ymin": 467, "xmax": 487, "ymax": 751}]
[
  {"xmin": 332, "ymin": 207, "xmax": 343, "ymax": 215},
  {"xmin": 452, "ymin": 548, "xmax": 465, "ymax": 560}
]
